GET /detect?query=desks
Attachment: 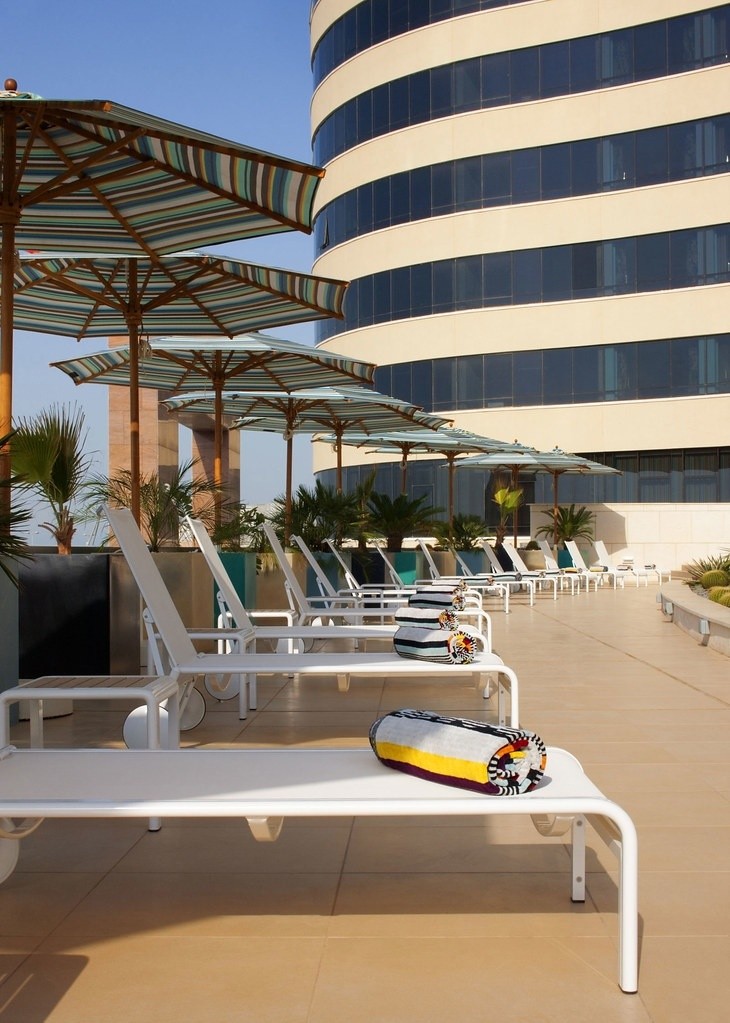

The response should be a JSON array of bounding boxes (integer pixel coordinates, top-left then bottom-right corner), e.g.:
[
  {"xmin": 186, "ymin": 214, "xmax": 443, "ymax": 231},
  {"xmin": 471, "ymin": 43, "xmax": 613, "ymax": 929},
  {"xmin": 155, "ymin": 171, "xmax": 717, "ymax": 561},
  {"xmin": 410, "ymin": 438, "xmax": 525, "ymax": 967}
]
[
  {"xmin": 360, "ymin": 584, "xmax": 400, "ymax": 608},
  {"xmin": 305, "ymin": 596, "xmax": 363, "ymax": 648},
  {"xmin": 337, "ymin": 589, "xmax": 384, "ymax": 625},
  {"xmin": 1, "ymin": 676, "xmax": 180, "ymax": 831},
  {"xmin": 217, "ymin": 609, "xmax": 299, "ymax": 678},
  {"xmin": 147, "ymin": 628, "xmax": 258, "ymax": 721}
]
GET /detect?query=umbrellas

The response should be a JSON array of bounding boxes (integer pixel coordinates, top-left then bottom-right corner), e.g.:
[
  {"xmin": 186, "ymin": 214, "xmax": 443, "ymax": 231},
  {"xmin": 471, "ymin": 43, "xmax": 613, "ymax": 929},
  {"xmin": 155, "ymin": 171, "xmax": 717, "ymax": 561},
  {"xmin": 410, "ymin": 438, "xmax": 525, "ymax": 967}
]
[
  {"xmin": 162, "ymin": 381, "xmax": 420, "ymax": 542},
  {"xmin": 0, "ymin": 78, "xmax": 326, "ymax": 543},
  {"xmin": 52, "ymin": 329, "xmax": 373, "ymax": 538},
  {"xmin": 12, "ymin": 252, "xmax": 347, "ymax": 543},
  {"xmin": 237, "ymin": 414, "xmax": 624, "ymax": 548}
]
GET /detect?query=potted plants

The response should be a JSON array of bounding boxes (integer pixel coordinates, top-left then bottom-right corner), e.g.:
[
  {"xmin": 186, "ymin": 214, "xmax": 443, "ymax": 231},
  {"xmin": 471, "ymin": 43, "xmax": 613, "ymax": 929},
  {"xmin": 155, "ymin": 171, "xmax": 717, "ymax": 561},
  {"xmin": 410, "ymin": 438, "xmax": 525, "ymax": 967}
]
[
  {"xmin": 518, "ymin": 541, "xmax": 558, "ymax": 571},
  {"xmin": 2, "ymin": 403, "xmax": 458, "ymax": 726},
  {"xmin": 534, "ymin": 504, "xmax": 598, "ymax": 568},
  {"xmin": 450, "ymin": 509, "xmax": 491, "ymax": 577},
  {"xmin": 489, "ymin": 470, "xmax": 526, "ymax": 573}
]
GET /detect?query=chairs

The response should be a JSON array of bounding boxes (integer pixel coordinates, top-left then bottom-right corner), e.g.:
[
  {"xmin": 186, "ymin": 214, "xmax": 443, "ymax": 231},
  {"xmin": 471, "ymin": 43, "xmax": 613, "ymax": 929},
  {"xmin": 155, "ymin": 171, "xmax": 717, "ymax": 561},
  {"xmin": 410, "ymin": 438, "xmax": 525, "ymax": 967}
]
[{"xmin": 0, "ymin": 508, "xmax": 672, "ymax": 992}]
